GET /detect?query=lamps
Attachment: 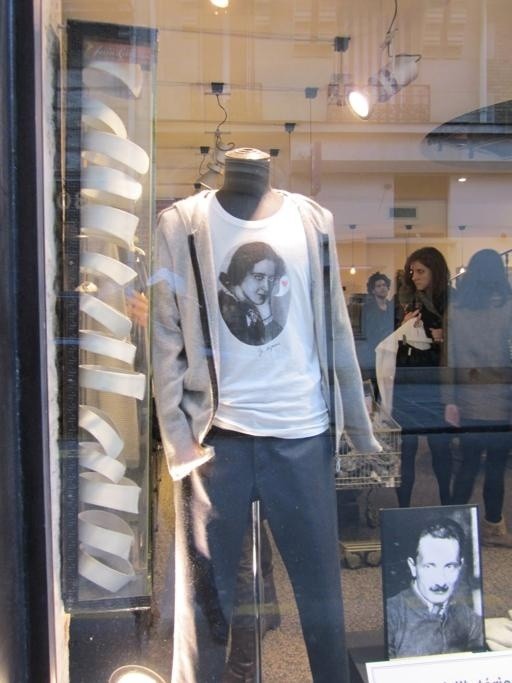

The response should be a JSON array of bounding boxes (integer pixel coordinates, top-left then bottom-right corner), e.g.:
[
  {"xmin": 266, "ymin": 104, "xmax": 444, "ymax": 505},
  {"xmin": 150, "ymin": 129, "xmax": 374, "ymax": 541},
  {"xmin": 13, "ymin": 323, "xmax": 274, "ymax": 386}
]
[
  {"xmin": 195, "ymin": 143, "xmax": 230, "ymax": 190},
  {"xmin": 350, "ymin": 225, "xmax": 358, "ymax": 274},
  {"xmin": 459, "ymin": 226, "xmax": 467, "ymax": 273},
  {"xmin": 208, "ymin": 82, "xmax": 235, "ymax": 173},
  {"xmin": 347, "ymin": 0, "xmax": 423, "ymax": 117}
]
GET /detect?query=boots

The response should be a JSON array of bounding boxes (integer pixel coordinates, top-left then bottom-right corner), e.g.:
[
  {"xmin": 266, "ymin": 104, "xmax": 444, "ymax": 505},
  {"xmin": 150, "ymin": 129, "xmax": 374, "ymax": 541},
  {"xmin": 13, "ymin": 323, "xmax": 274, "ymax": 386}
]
[{"xmin": 479, "ymin": 514, "xmax": 512, "ymax": 547}]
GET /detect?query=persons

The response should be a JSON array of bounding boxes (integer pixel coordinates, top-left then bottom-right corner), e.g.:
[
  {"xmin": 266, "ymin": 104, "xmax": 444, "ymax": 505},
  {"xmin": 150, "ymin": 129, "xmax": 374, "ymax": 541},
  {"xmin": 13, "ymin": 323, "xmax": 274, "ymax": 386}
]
[
  {"xmin": 151, "ymin": 147, "xmax": 382, "ymax": 683},
  {"xmin": 360, "ymin": 270, "xmax": 394, "ymax": 413},
  {"xmin": 372, "ymin": 248, "xmax": 456, "ymax": 507},
  {"xmin": 438, "ymin": 250, "xmax": 511, "ymax": 545},
  {"xmin": 219, "ymin": 243, "xmax": 285, "ymax": 344}
]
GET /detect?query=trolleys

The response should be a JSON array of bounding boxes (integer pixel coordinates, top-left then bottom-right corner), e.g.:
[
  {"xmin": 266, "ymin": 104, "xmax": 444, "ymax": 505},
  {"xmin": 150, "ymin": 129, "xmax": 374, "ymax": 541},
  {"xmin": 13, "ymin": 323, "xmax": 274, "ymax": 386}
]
[{"xmin": 330, "ymin": 378, "xmax": 402, "ymax": 569}]
